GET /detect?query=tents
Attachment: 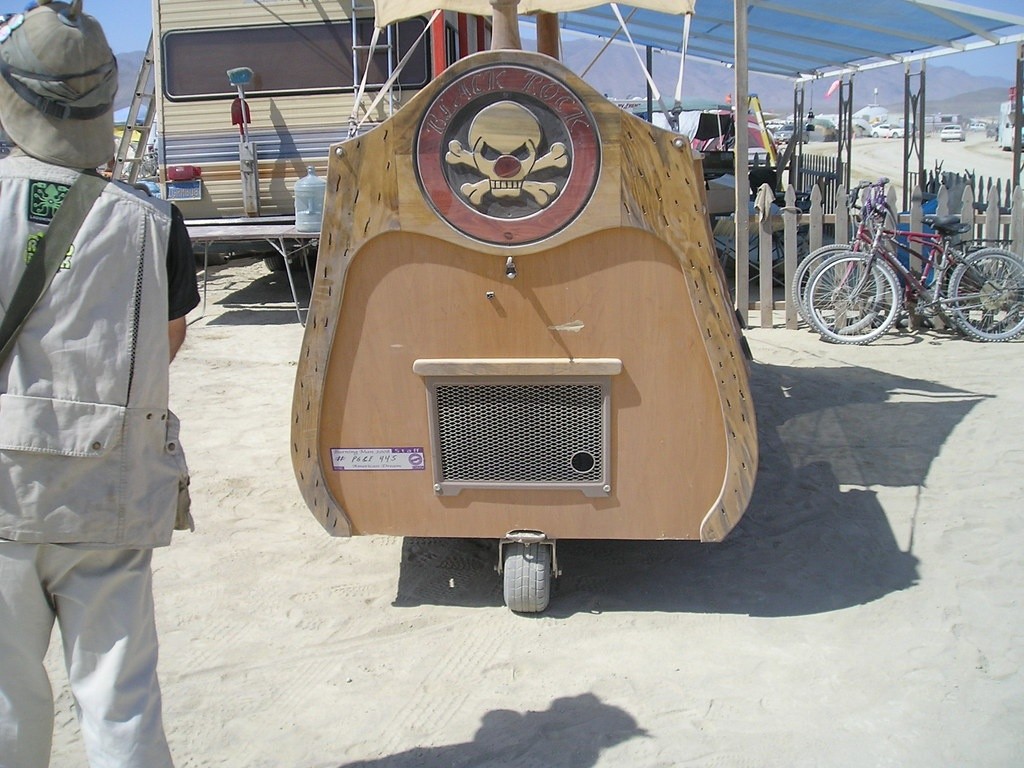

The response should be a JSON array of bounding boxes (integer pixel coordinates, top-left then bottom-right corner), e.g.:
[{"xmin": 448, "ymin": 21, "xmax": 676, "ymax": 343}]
[{"xmin": 420, "ymin": 0, "xmax": 1022, "ymax": 336}]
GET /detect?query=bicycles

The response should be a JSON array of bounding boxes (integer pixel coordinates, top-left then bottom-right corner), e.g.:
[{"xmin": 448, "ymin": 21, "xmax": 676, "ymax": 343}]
[{"xmin": 791, "ymin": 176, "xmax": 1024, "ymax": 346}]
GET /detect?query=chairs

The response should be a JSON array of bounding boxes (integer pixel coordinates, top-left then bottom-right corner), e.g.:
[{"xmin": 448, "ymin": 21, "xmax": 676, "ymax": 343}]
[{"xmin": 713, "ymin": 229, "xmax": 808, "ymax": 293}]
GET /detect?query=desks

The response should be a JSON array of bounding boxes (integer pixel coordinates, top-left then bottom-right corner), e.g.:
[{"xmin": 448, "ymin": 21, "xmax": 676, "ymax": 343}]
[{"xmin": 184, "ymin": 225, "xmax": 320, "ymax": 328}]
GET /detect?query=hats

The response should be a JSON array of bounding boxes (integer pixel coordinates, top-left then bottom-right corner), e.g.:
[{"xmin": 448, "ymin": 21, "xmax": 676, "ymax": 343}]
[{"xmin": 0, "ymin": 0, "xmax": 118, "ymax": 169}]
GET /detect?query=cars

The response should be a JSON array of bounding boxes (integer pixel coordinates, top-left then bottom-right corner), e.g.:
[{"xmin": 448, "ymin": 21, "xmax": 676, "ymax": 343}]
[
  {"xmin": 970, "ymin": 122, "xmax": 987, "ymax": 132},
  {"xmin": 939, "ymin": 124, "xmax": 966, "ymax": 142},
  {"xmin": 773, "ymin": 123, "xmax": 810, "ymax": 145},
  {"xmin": 869, "ymin": 124, "xmax": 912, "ymax": 139}
]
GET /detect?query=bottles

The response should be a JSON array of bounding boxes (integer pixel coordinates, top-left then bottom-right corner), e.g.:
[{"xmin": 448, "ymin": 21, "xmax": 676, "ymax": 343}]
[{"xmin": 294, "ymin": 166, "xmax": 328, "ymax": 233}]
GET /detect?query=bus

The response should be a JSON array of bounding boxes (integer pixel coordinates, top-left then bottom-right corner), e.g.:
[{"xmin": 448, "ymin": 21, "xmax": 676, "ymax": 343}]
[{"xmin": 145, "ymin": 0, "xmax": 494, "ymax": 271}]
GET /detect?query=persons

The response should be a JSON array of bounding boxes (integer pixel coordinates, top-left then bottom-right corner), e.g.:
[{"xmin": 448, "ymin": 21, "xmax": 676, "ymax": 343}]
[{"xmin": 1, "ymin": 5, "xmax": 201, "ymax": 767}]
